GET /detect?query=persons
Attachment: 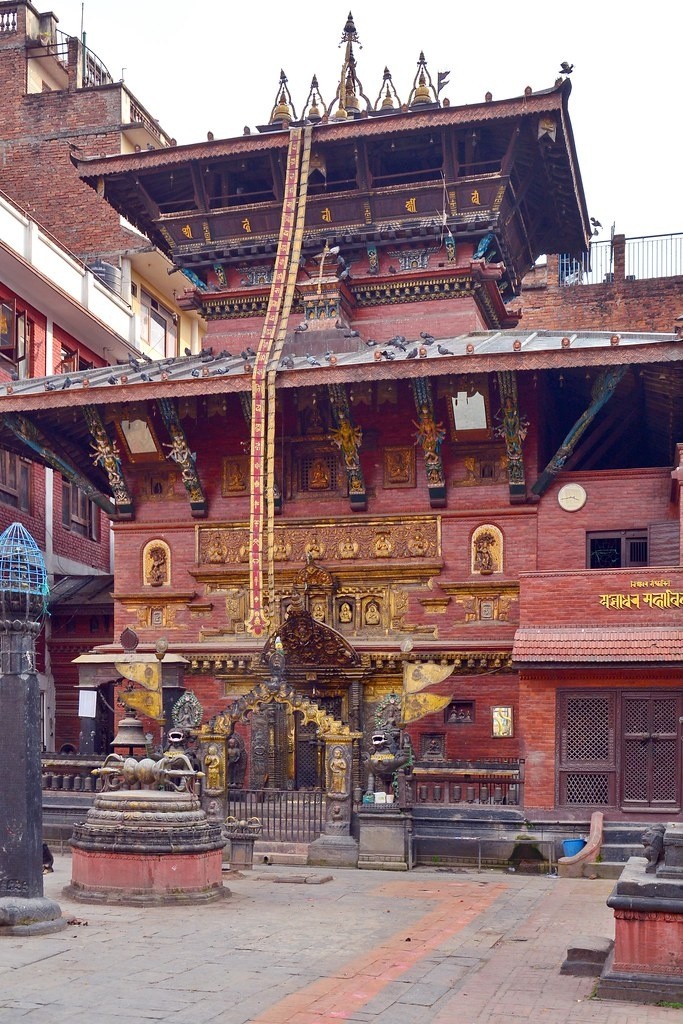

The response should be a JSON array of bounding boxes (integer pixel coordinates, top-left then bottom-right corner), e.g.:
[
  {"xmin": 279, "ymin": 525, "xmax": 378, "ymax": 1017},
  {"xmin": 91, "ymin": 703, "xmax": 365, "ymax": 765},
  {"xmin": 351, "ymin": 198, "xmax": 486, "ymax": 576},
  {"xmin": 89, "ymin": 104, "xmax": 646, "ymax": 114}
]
[
  {"xmin": 377, "ymin": 537, "xmax": 389, "ymax": 557},
  {"xmin": 210, "ymin": 542, "xmax": 222, "ymax": 562},
  {"xmin": 42, "ymin": 843, "xmax": 54, "ymax": 873},
  {"xmin": 228, "ymin": 464, "xmax": 243, "ymax": 486},
  {"xmin": 341, "ymin": 607, "xmax": 350, "ymax": 620},
  {"xmin": 411, "ymin": 417, "xmax": 446, "ymax": 451},
  {"xmin": 450, "ymin": 706, "xmax": 471, "ymax": 720},
  {"xmin": 368, "ymin": 608, "xmax": 377, "ymax": 622},
  {"xmin": 205, "ymin": 745, "xmax": 221, "ymax": 790},
  {"xmin": 349, "ymin": 476, "xmax": 363, "ymax": 494},
  {"xmin": 315, "ymin": 607, "xmax": 322, "ymax": 618},
  {"xmin": 242, "ymin": 542, "xmax": 249, "ymax": 558},
  {"xmin": 330, "ymin": 748, "xmax": 347, "ymax": 794},
  {"xmin": 151, "ymin": 555, "xmax": 164, "ymax": 581},
  {"xmin": 228, "ymin": 739, "xmax": 241, "ymax": 784},
  {"xmin": 162, "ymin": 436, "xmax": 192, "ymax": 468},
  {"xmin": 391, "ymin": 456, "xmax": 407, "ymax": 477},
  {"xmin": 492, "ymin": 410, "xmax": 531, "ymax": 455},
  {"xmin": 178, "ymin": 707, "xmax": 193, "ymax": 726},
  {"xmin": 89, "ymin": 440, "xmax": 121, "ymax": 481},
  {"xmin": 412, "ymin": 536, "xmax": 423, "ymax": 556},
  {"xmin": 307, "ymin": 540, "xmax": 319, "ymax": 559},
  {"xmin": 312, "ymin": 465, "xmax": 326, "ymax": 483},
  {"xmin": 430, "ymin": 470, "xmax": 440, "ymax": 484},
  {"xmin": 341, "ymin": 538, "xmax": 354, "ymax": 557},
  {"xmin": 481, "ymin": 542, "xmax": 490, "ymax": 570},
  {"xmin": 275, "ymin": 540, "xmax": 288, "ymax": 560},
  {"xmin": 328, "ymin": 421, "xmax": 362, "ymax": 468}
]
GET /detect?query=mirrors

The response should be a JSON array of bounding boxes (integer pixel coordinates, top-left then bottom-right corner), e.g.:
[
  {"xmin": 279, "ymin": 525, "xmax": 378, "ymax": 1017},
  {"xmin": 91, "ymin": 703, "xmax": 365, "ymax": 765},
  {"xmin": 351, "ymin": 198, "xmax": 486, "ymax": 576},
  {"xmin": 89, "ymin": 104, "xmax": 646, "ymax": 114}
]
[
  {"xmin": 445, "ymin": 373, "xmax": 493, "ymax": 444},
  {"xmin": 114, "ymin": 406, "xmax": 165, "ymax": 465}
]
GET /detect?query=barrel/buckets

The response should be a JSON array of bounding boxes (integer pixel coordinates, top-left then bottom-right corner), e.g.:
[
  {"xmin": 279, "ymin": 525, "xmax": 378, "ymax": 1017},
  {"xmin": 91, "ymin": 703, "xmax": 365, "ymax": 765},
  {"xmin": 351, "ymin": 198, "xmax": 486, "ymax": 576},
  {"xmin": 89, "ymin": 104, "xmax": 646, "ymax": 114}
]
[
  {"xmin": 373, "ymin": 792, "xmax": 385, "ymax": 803},
  {"xmin": 561, "ymin": 838, "xmax": 585, "ymax": 857}
]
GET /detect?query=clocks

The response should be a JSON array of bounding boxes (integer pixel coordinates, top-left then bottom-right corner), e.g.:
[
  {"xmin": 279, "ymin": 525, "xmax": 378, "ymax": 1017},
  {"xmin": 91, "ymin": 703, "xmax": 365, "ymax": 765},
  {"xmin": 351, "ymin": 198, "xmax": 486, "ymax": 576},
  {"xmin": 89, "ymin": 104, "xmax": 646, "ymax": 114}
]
[{"xmin": 558, "ymin": 482, "xmax": 587, "ymax": 512}]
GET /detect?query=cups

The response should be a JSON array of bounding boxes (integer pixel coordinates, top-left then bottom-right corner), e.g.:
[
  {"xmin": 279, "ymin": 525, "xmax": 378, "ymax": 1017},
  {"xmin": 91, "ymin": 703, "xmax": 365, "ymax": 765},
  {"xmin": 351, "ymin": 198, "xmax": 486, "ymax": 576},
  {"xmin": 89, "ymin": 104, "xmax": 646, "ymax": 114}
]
[{"xmin": 386, "ymin": 795, "xmax": 393, "ymax": 803}]
